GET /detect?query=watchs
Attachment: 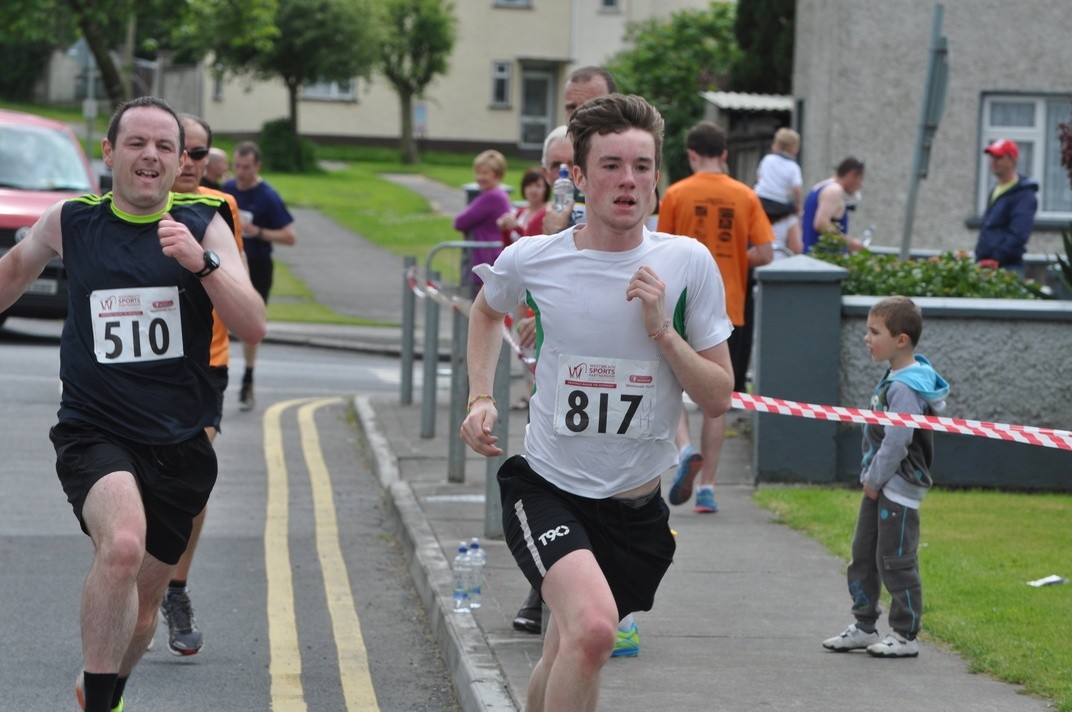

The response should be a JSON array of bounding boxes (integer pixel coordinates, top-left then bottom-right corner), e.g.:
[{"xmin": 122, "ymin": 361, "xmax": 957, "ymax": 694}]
[{"xmin": 194, "ymin": 250, "xmax": 220, "ymax": 277}]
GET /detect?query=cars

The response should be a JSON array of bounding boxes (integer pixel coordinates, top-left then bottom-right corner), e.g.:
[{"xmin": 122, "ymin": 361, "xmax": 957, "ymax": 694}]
[{"xmin": 0, "ymin": 106, "xmax": 99, "ymax": 331}]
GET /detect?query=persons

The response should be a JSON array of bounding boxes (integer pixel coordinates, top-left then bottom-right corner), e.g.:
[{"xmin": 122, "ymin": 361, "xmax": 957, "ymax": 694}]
[
  {"xmin": 220, "ymin": 141, "xmax": 298, "ymax": 413},
  {"xmin": 819, "ymin": 296, "xmax": 953, "ymax": 660},
  {"xmin": 459, "ymin": 92, "xmax": 737, "ymax": 710},
  {"xmin": 976, "ymin": 137, "xmax": 1040, "ymax": 273},
  {"xmin": 456, "ymin": 66, "xmax": 867, "ymax": 660},
  {"xmin": 198, "ymin": 147, "xmax": 231, "ymax": 189},
  {"xmin": 0, "ymin": 96, "xmax": 266, "ymax": 712},
  {"xmin": 144, "ymin": 112, "xmax": 250, "ymax": 655}
]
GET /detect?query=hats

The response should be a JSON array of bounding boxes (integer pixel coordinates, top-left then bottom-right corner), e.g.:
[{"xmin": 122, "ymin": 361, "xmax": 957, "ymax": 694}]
[{"xmin": 984, "ymin": 138, "xmax": 1018, "ymax": 161}]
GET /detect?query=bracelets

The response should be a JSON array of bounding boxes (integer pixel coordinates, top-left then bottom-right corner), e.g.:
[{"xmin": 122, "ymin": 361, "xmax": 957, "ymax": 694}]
[{"xmin": 466, "ymin": 393, "xmax": 496, "ymax": 414}]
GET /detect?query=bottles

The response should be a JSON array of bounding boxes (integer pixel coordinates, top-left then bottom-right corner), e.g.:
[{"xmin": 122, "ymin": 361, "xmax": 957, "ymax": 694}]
[
  {"xmin": 553, "ymin": 164, "xmax": 574, "ymax": 212},
  {"xmin": 452, "ymin": 538, "xmax": 472, "ymax": 615},
  {"xmin": 863, "ymin": 224, "xmax": 875, "ymax": 246},
  {"xmin": 468, "ymin": 537, "xmax": 488, "ymax": 608}
]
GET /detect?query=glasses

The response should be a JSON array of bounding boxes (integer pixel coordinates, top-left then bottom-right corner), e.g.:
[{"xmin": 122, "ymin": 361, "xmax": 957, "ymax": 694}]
[{"xmin": 182, "ymin": 147, "xmax": 207, "ymax": 160}]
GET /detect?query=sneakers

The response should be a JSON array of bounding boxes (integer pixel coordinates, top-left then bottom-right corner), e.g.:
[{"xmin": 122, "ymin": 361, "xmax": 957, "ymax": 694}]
[
  {"xmin": 609, "ymin": 623, "xmax": 639, "ymax": 658},
  {"xmin": 75, "ymin": 667, "xmax": 124, "ymax": 712},
  {"xmin": 693, "ymin": 483, "xmax": 718, "ymax": 514},
  {"xmin": 669, "ymin": 447, "xmax": 703, "ymax": 505},
  {"xmin": 821, "ymin": 623, "xmax": 880, "ymax": 652},
  {"xmin": 866, "ymin": 631, "xmax": 920, "ymax": 658},
  {"xmin": 240, "ymin": 380, "xmax": 255, "ymax": 409},
  {"xmin": 147, "ymin": 586, "xmax": 204, "ymax": 656},
  {"xmin": 514, "ymin": 587, "xmax": 545, "ymax": 632}
]
